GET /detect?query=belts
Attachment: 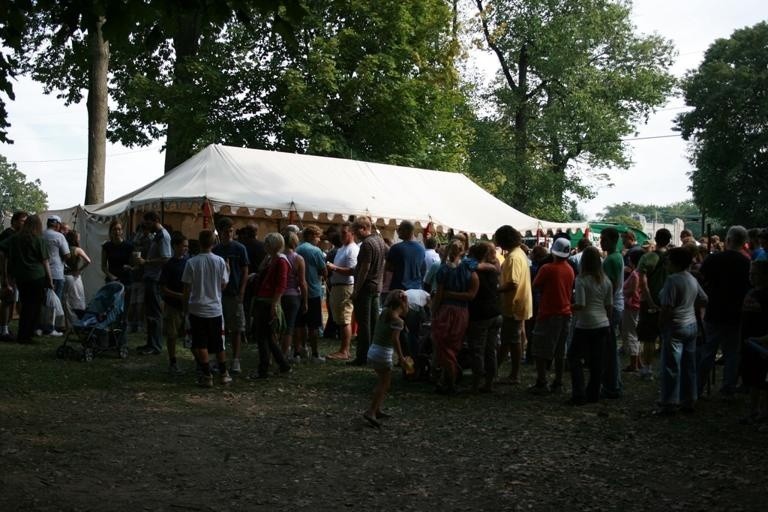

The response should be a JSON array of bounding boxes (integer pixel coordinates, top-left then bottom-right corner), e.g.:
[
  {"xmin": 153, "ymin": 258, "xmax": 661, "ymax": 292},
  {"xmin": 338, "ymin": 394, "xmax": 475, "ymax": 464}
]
[{"xmin": 331, "ymin": 283, "xmax": 354, "ymax": 286}]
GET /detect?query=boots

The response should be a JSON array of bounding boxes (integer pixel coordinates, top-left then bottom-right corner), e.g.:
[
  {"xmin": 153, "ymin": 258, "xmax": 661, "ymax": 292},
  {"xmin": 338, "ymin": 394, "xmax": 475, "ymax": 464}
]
[{"xmin": 563, "ymin": 387, "xmax": 620, "ymax": 407}]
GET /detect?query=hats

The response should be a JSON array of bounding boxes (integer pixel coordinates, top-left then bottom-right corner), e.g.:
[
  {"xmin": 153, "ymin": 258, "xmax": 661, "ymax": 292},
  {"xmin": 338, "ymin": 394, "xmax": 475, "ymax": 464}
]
[
  {"xmin": 551, "ymin": 238, "xmax": 571, "ymax": 259},
  {"xmin": 47, "ymin": 215, "xmax": 62, "ymax": 225}
]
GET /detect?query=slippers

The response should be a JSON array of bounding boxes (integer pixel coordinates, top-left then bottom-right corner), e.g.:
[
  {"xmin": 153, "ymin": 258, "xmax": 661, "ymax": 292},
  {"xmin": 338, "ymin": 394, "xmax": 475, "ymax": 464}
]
[{"xmin": 325, "ymin": 351, "xmax": 348, "ymax": 360}]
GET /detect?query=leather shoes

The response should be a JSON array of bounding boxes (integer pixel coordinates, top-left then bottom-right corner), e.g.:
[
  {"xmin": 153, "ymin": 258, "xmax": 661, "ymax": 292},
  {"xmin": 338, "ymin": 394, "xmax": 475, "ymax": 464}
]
[{"xmin": 136, "ymin": 344, "xmax": 160, "ymax": 355}]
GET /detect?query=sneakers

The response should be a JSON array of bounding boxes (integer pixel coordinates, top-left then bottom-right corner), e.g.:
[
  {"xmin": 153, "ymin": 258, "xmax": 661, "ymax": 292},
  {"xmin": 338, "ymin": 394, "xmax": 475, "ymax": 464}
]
[
  {"xmin": 499, "ymin": 376, "xmax": 521, "ymax": 384},
  {"xmin": 220, "ymin": 369, "xmax": 233, "ymax": 383},
  {"xmin": 654, "ymin": 378, "xmax": 756, "ymax": 421},
  {"xmin": 0, "ymin": 329, "xmax": 16, "ymax": 342},
  {"xmin": 528, "ymin": 383, "xmax": 550, "ymax": 395},
  {"xmin": 362, "ymin": 411, "xmax": 378, "ymax": 427},
  {"xmin": 540, "ymin": 393, "xmax": 561, "ymax": 402},
  {"xmin": 622, "ymin": 362, "xmax": 644, "ymax": 372},
  {"xmin": 245, "ymin": 372, "xmax": 269, "ymax": 380},
  {"xmin": 49, "ymin": 329, "xmax": 63, "ymax": 337},
  {"xmin": 168, "ymin": 361, "xmax": 186, "ymax": 375},
  {"xmin": 204, "ymin": 372, "xmax": 214, "ymax": 387},
  {"xmin": 310, "ymin": 355, "xmax": 326, "ymax": 363},
  {"xmin": 478, "ymin": 383, "xmax": 492, "ymax": 393},
  {"xmin": 231, "ymin": 357, "xmax": 241, "ymax": 372},
  {"xmin": 36, "ymin": 328, "xmax": 43, "ymax": 336},
  {"xmin": 376, "ymin": 410, "xmax": 392, "ymax": 419}
]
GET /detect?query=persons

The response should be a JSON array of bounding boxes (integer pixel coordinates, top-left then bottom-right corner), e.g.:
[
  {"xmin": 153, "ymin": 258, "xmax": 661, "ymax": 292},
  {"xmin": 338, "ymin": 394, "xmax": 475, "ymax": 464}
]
[
  {"xmin": 1, "ymin": 209, "xmax": 92, "ymax": 344},
  {"xmin": 364, "ymin": 289, "xmax": 409, "ymax": 428},
  {"xmin": 101, "ymin": 209, "xmax": 768, "ymax": 407}
]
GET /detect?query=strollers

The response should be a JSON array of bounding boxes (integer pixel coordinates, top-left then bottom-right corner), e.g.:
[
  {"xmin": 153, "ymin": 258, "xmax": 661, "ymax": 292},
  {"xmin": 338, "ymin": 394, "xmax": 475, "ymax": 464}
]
[
  {"xmin": 57, "ymin": 278, "xmax": 129, "ymax": 363},
  {"xmin": 404, "ymin": 290, "xmax": 435, "ymax": 386}
]
